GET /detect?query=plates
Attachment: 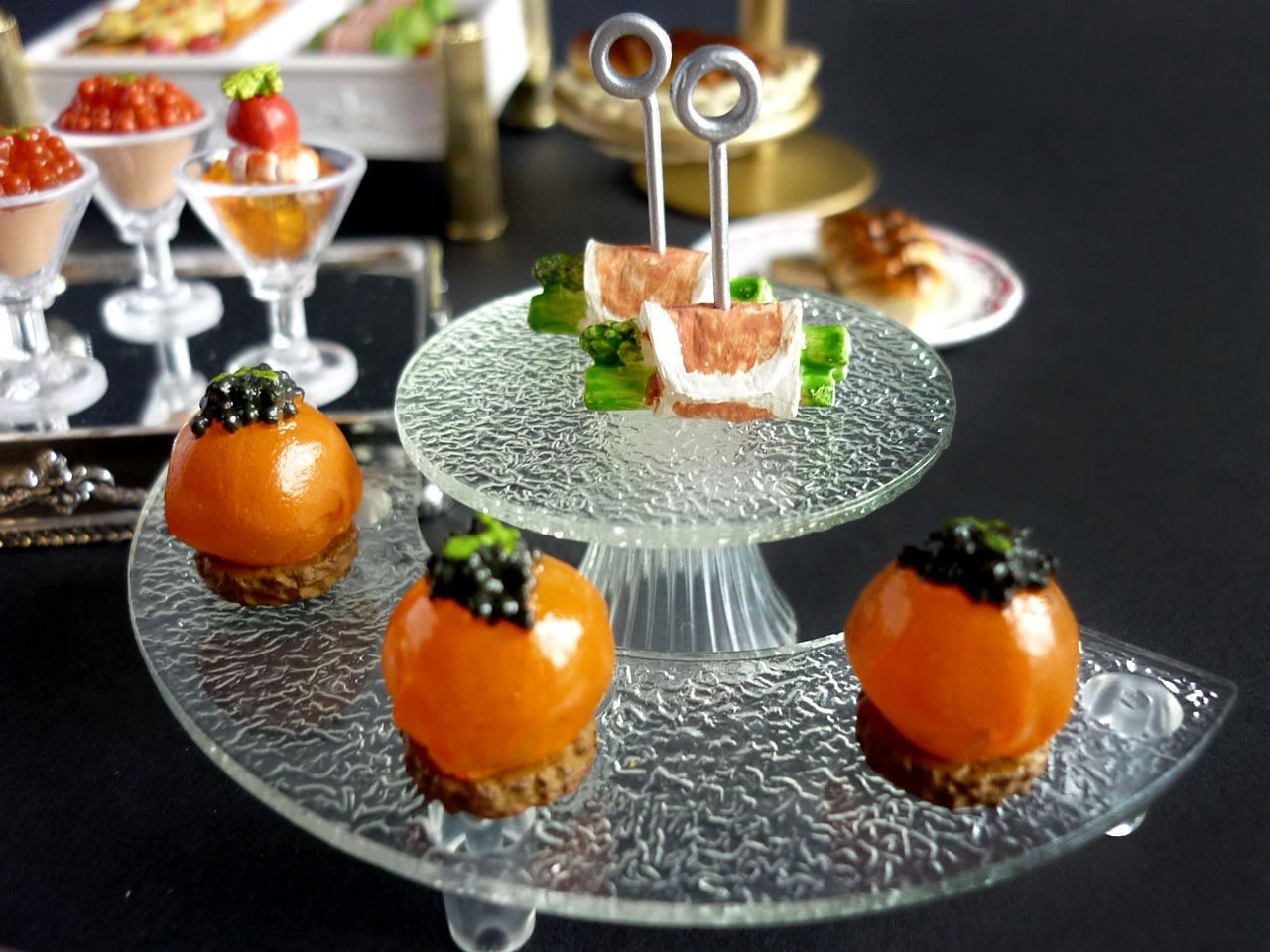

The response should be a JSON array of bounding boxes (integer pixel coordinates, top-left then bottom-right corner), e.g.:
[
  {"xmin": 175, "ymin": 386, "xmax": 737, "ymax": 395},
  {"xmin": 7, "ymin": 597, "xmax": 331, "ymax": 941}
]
[
  {"xmin": 16, "ymin": 0, "xmax": 533, "ymax": 166},
  {"xmin": 542, "ymin": 78, "xmax": 822, "ymax": 159},
  {"xmin": 686, "ymin": 210, "xmax": 1022, "ymax": 351}
]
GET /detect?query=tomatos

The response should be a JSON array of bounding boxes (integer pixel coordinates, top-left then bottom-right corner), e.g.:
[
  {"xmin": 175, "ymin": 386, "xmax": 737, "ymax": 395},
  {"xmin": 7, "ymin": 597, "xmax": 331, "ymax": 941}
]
[{"xmin": 0, "ymin": 73, "xmax": 299, "ymax": 217}]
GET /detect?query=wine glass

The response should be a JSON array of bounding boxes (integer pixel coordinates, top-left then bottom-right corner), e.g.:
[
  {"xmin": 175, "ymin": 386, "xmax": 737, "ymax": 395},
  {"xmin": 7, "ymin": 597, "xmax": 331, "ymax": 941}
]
[
  {"xmin": 176, "ymin": 139, "xmax": 369, "ymax": 411},
  {"xmin": 48, "ymin": 106, "xmax": 225, "ymax": 344},
  {"xmin": 0, "ymin": 151, "xmax": 109, "ymax": 428}
]
